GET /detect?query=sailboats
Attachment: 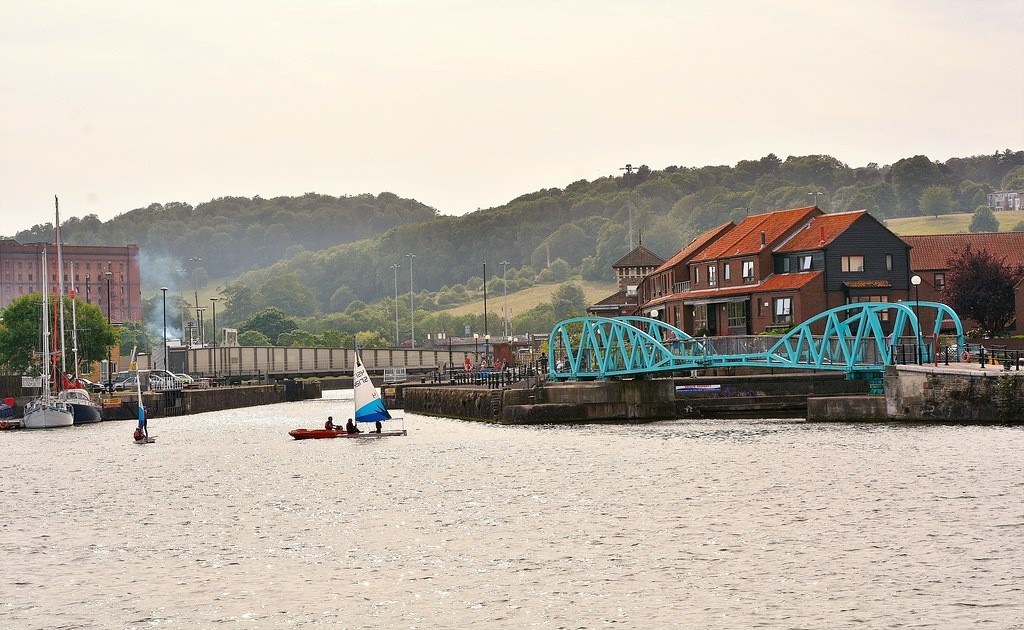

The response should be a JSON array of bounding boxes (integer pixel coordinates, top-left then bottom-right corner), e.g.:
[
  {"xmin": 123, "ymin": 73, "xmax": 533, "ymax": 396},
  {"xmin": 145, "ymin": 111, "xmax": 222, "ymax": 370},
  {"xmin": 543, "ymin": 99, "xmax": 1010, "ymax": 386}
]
[
  {"xmin": 337, "ymin": 334, "xmax": 408, "ymax": 437},
  {"xmin": 23, "ymin": 193, "xmax": 103, "ymax": 428}
]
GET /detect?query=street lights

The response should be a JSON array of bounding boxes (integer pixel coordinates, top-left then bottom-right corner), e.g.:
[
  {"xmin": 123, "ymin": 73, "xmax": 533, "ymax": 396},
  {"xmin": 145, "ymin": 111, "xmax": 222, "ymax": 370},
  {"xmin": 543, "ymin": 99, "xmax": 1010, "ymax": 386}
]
[
  {"xmin": 500, "ymin": 261, "xmax": 511, "ymax": 336},
  {"xmin": 105, "ymin": 271, "xmax": 114, "ymax": 393},
  {"xmin": 650, "ymin": 310, "xmax": 659, "ymax": 340},
  {"xmin": 513, "ymin": 338, "xmax": 518, "ymax": 382},
  {"xmin": 558, "ymin": 328, "xmax": 563, "ymax": 372},
  {"xmin": 597, "ymin": 329, "xmax": 601, "ymax": 355},
  {"xmin": 405, "ymin": 252, "xmax": 417, "ymax": 348},
  {"xmin": 911, "ymin": 274, "xmax": 923, "ymax": 365},
  {"xmin": 485, "ymin": 335, "xmax": 490, "ymax": 372},
  {"xmin": 506, "ymin": 336, "xmax": 513, "ymax": 385},
  {"xmin": 391, "ymin": 264, "xmax": 401, "ymax": 345},
  {"xmin": 474, "ymin": 334, "xmax": 480, "ymax": 368},
  {"xmin": 160, "ymin": 287, "xmax": 168, "ymax": 378},
  {"xmin": 210, "ymin": 297, "xmax": 219, "ymax": 387}
]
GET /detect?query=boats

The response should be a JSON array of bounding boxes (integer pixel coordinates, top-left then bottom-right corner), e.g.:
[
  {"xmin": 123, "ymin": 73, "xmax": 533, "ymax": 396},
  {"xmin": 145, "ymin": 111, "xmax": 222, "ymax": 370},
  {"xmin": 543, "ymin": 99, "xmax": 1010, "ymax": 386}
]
[
  {"xmin": 0, "ymin": 398, "xmax": 24, "ymax": 430},
  {"xmin": 287, "ymin": 429, "xmax": 358, "ymax": 439}
]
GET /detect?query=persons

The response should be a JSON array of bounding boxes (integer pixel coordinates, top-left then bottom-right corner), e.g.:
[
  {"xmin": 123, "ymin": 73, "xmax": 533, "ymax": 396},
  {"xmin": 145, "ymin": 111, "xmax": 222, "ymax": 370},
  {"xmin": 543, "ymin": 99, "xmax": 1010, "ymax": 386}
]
[
  {"xmin": 689, "ymin": 350, "xmax": 698, "ymax": 378},
  {"xmin": 502, "ymin": 357, "xmax": 508, "ymax": 372},
  {"xmin": 143, "ymin": 406, "xmax": 148, "ymax": 439},
  {"xmin": 325, "ymin": 417, "xmax": 336, "ymax": 430},
  {"xmin": 539, "ymin": 352, "xmax": 548, "ymax": 374},
  {"xmin": 556, "ymin": 358, "xmax": 564, "ymax": 374},
  {"xmin": 134, "ymin": 428, "xmax": 148, "ymax": 441},
  {"xmin": 886, "ymin": 333, "xmax": 897, "ymax": 361},
  {"xmin": 666, "ymin": 331, "xmax": 683, "ymax": 365},
  {"xmin": 494, "ymin": 357, "xmax": 502, "ymax": 371},
  {"xmin": 346, "ymin": 418, "xmax": 359, "ymax": 435}
]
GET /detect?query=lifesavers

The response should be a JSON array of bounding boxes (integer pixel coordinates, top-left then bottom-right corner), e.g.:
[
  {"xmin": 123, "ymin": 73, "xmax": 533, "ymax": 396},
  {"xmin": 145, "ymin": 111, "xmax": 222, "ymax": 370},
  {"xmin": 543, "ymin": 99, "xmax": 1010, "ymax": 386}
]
[{"xmin": 960, "ymin": 351, "xmax": 969, "ymax": 362}]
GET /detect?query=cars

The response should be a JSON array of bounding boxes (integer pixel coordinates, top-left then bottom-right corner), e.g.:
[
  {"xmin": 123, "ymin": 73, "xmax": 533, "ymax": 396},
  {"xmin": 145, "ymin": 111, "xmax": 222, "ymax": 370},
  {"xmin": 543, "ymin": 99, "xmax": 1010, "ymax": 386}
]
[
  {"xmin": 106, "ymin": 369, "xmax": 195, "ymax": 391},
  {"xmin": 400, "ymin": 340, "xmax": 416, "ymax": 347},
  {"xmin": 936, "ymin": 344, "xmax": 991, "ymax": 364},
  {"xmin": 73, "ymin": 378, "xmax": 104, "ymax": 394}
]
